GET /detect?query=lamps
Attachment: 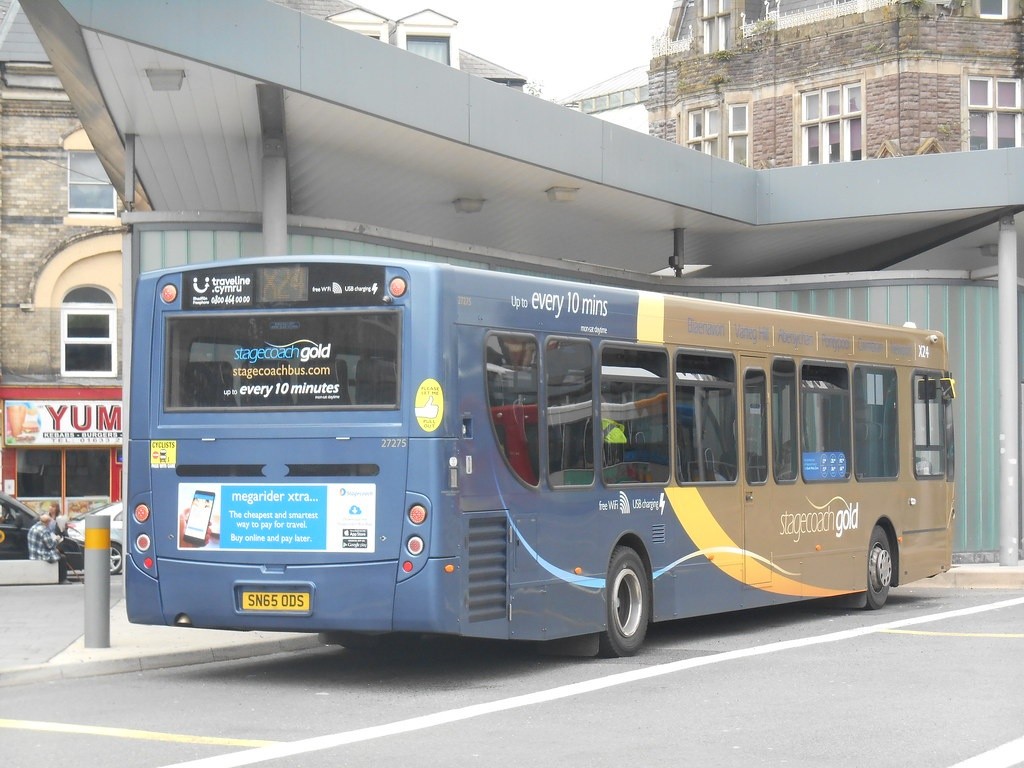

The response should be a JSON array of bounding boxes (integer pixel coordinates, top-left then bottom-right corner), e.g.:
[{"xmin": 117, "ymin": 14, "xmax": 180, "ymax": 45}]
[
  {"xmin": 453, "ymin": 198, "xmax": 487, "ymax": 215},
  {"xmin": 545, "ymin": 187, "xmax": 580, "ymax": 202},
  {"xmin": 146, "ymin": 69, "xmax": 186, "ymax": 92},
  {"xmin": 979, "ymin": 244, "xmax": 998, "ymax": 256}
]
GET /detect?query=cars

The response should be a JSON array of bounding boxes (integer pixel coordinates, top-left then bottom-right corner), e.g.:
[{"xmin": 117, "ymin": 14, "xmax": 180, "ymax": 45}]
[
  {"xmin": 61, "ymin": 502, "xmax": 125, "ymax": 575},
  {"xmin": 0, "ymin": 492, "xmax": 82, "ymax": 582}
]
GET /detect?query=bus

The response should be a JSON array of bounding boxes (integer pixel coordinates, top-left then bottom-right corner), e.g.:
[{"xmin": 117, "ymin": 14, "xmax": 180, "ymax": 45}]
[{"xmin": 125, "ymin": 254, "xmax": 955, "ymax": 659}]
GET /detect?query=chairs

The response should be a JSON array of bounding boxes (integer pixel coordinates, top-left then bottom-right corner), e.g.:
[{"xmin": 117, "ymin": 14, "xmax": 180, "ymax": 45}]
[{"xmin": 179, "ymin": 356, "xmax": 396, "ymax": 408}]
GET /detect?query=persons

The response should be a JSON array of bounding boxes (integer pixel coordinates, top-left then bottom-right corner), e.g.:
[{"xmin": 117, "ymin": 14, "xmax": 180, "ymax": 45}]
[
  {"xmin": 776, "ymin": 441, "xmax": 795, "ymax": 477},
  {"xmin": 26, "ymin": 500, "xmax": 72, "ymax": 587},
  {"xmin": 594, "ymin": 393, "xmax": 630, "ymax": 464}
]
